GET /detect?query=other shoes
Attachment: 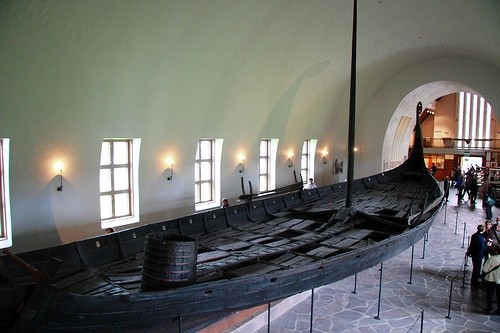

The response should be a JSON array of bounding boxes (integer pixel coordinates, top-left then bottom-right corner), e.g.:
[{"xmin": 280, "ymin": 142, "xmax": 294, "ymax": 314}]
[
  {"xmin": 484, "ymin": 308, "xmax": 500, "ymax": 315},
  {"xmin": 485, "ymin": 214, "xmax": 493, "ymax": 220},
  {"xmin": 443, "ymin": 199, "xmax": 445, "ymax": 201},
  {"xmin": 457, "ymin": 201, "xmax": 463, "ymax": 206},
  {"xmin": 471, "ymin": 281, "xmax": 480, "ymax": 288},
  {"xmin": 470, "ymin": 201, "xmax": 476, "ymax": 204},
  {"xmin": 445, "ymin": 199, "xmax": 450, "ymax": 202}
]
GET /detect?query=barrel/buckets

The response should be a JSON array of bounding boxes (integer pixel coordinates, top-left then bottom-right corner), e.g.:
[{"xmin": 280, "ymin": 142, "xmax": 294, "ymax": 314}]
[{"xmin": 140, "ymin": 232, "xmax": 197, "ymax": 295}]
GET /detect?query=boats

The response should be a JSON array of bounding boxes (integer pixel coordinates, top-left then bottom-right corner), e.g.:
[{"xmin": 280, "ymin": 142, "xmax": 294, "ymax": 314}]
[{"xmin": 0, "ymin": 100, "xmax": 450, "ymax": 333}]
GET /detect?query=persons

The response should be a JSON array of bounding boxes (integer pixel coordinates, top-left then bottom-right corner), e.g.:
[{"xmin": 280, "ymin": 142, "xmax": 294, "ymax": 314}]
[
  {"xmin": 335, "ymin": 158, "xmax": 340, "ymax": 174},
  {"xmin": 105, "ymin": 227, "xmax": 114, "ymax": 234},
  {"xmin": 466, "ymin": 217, "xmax": 500, "ymax": 315},
  {"xmin": 307, "ymin": 178, "xmax": 318, "ymax": 189},
  {"xmin": 430, "ymin": 163, "xmax": 497, "ymax": 220},
  {"xmin": 223, "ymin": 199, "xmax": 229, "ymax": 207}
]
